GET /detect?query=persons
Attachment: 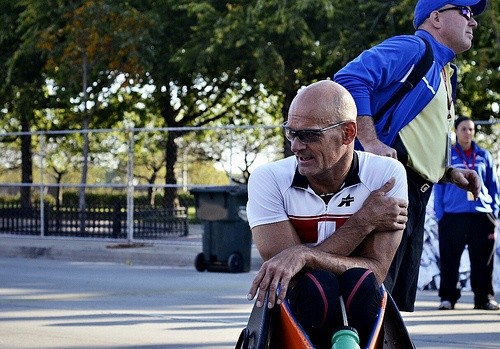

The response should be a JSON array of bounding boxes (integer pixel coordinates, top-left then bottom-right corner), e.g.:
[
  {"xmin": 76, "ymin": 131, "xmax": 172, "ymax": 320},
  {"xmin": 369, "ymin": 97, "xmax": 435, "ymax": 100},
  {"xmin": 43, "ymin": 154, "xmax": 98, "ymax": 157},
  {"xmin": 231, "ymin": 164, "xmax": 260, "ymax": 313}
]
[
  {"xmin": 334, "ymin": 0, "xmax": 480, "ymax": 311},
  {"xmin": 246, "ymin": 80, "xmax": 409, "ymax": 308},
  {"xmin": 434, "ymin": 116, "xmax": 500, "ymax": 310}
]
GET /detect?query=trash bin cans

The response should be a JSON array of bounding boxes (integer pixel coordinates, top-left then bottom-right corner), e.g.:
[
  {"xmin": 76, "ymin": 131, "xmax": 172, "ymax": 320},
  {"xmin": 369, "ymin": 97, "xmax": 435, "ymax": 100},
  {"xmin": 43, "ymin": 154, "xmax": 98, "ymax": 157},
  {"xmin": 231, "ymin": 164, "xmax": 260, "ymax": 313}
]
[{"xmin": 188, "ymin": 184, "xmax": 253, "ymax": 275}]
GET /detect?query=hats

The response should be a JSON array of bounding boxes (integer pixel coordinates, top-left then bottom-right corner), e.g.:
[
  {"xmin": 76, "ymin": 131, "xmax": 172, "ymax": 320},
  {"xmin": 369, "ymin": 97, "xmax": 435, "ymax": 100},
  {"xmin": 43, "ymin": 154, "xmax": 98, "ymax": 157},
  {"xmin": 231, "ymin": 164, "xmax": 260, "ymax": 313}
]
[{"xmin": 413, "ymin": 0, "xmax": 479, "ymax": 29}]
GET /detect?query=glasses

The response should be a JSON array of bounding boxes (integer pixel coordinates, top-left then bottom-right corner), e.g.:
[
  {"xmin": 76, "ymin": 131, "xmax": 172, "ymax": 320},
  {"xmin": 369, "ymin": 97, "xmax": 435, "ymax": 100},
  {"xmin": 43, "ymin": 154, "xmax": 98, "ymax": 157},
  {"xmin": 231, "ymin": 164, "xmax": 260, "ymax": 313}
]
[
  {"xmin": 428, "ymin": 5, "xmax": 474, "ymax": 21},
  {"xmin": 280, "ymin": 118, "xmax": 344, "ymax": 144}
]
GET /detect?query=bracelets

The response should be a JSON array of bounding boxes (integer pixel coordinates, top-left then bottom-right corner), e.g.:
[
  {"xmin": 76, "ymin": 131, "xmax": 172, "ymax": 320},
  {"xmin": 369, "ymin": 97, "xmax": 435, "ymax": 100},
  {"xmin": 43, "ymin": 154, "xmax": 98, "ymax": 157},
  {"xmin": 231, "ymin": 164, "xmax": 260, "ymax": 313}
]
[{"xmin": 446, "ymin": 168, "xmax": 455, "ymax": 184}]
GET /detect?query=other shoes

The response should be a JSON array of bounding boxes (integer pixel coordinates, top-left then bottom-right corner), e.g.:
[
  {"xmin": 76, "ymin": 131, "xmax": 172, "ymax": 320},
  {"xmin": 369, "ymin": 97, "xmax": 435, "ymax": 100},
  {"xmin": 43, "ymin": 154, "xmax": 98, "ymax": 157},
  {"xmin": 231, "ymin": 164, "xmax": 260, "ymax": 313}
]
[
  {"xmin": 439, "ymin": 300, "xmax": 455, "ymax": 310},
  {"xmin": 472, "ymin": 299, "xmax": 500, "ymax": 310}
]
